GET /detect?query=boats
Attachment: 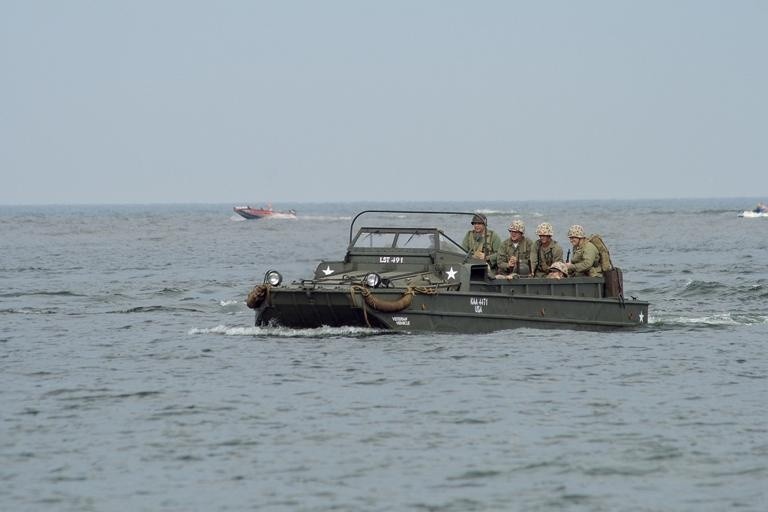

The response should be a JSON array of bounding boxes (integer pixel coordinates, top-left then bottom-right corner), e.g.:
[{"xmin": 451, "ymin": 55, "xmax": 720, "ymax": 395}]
[
  {"xmin": 245, "ymin": 210, "xmax": 651, "ymax": 337},
  {"xmin": 737, "ymin": 209, "xmax": 767, "ymax": 219},
  {"xmin": 233, "ymin": 206, "xmax": 297, "ymax": 220}
]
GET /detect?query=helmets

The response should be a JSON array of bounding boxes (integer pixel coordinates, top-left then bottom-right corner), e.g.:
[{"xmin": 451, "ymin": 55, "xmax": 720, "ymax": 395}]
[
  {"xmin": 536, "ymin": 222, "xmax": 553, "ymax": 236},
  {"xmin": 507, "ymin": 220, "xmax": 525, "ymax": 234},
  {"xmin": 471, "ymin": 213, "xmax": 487, "ymax": 224},
  {"xmin": 568, "ymin": 224, "xmax": 586, "ymax": 238},
  {"xmin": 550, "ymin": 261, "xmax": 569, "ymax": 275}
]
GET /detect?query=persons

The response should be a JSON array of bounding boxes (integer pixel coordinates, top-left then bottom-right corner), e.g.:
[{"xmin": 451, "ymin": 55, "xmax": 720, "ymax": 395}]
[
  {"xmin": 529, "ymin": 223, "xmax": 563, "ymax": 279},
  {"xmin": 461, "ymin": 213, "xmax": 499, "ymax": 271},
  {"xmin": 496, "ymin": 220, "xmax": 534, "ymax": 279},
  {"xmin": 546, "ymin": 259, "xmax": 568, "ymax": 279},
  {"xmin": 565, "ymin": 224, "xmax": 606, "ymax": 277}
]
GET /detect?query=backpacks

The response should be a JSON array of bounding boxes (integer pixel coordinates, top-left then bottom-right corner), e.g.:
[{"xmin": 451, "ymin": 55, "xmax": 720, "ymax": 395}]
[{"xmin": 588, "ymin": 234, "xmax": 613, "ymax": 271}]
